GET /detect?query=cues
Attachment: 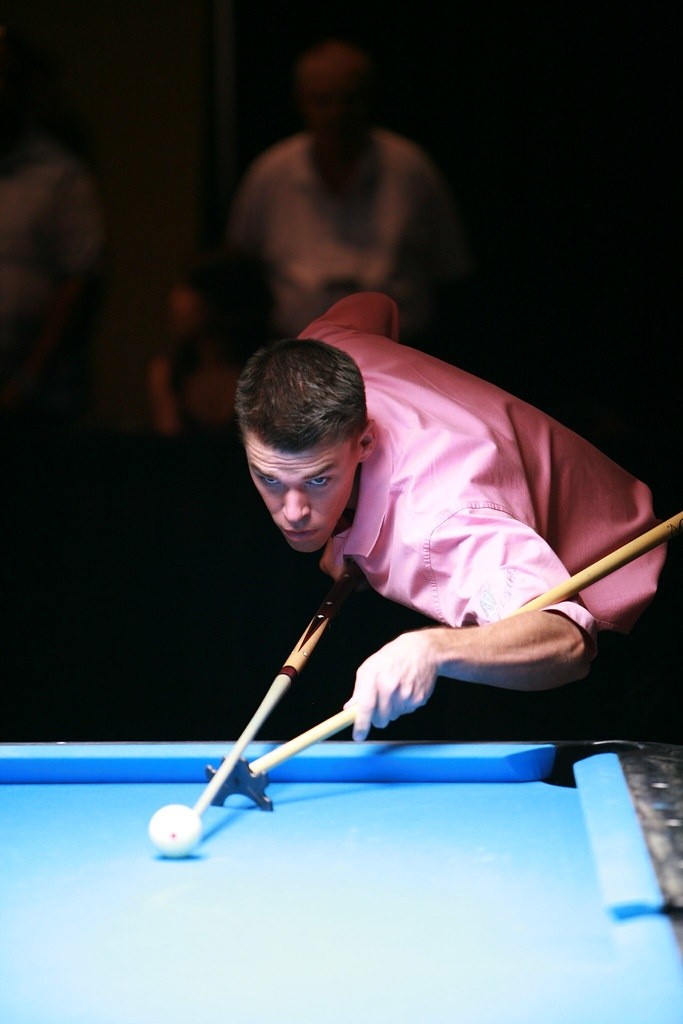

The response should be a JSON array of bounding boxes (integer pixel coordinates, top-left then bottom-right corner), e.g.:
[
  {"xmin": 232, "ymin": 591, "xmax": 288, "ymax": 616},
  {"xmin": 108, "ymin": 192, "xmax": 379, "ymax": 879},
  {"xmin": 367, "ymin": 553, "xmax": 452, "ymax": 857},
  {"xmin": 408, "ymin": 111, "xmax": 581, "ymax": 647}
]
[{"xmin": 193, "ymin": 556, "xmax": 362, "ymax": 818}]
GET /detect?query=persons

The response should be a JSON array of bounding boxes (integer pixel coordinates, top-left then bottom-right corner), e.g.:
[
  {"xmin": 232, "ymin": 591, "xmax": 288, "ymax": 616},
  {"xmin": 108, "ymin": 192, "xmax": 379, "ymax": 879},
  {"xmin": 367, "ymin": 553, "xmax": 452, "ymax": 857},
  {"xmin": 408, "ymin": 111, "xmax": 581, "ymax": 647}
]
[
  {"xmin": 234, "ymin": 291, "xmax": 683, "ymax": 745},
  {"xmin": 0, "ymin": 24, "xmax": 469, "ymax": 436}
]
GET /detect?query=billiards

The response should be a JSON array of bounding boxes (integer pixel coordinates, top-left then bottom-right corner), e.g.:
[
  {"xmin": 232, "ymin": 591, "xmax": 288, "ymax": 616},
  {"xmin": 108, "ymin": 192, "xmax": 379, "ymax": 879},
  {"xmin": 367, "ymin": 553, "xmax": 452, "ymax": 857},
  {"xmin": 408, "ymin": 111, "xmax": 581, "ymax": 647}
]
[{"xmin": 147, "ymin": 804, "xmax": 205, "ymax": 860}]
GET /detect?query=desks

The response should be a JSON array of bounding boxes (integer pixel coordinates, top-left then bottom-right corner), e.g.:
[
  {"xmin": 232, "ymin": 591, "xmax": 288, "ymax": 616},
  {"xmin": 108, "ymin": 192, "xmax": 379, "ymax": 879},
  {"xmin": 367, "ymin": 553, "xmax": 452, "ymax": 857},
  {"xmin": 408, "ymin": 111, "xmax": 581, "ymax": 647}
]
[{"xmin": 0, "ymin": 734, "xmax": 683, "ymax": 1024}]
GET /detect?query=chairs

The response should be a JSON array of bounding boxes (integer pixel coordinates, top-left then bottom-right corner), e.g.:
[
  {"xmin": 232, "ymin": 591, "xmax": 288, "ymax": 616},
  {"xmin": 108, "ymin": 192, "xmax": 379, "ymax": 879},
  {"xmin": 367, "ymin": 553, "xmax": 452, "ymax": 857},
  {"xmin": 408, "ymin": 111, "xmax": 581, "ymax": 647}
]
[{"xmin": 0, "ymin": 234, "xmax": 119, "ymax": 593}]
